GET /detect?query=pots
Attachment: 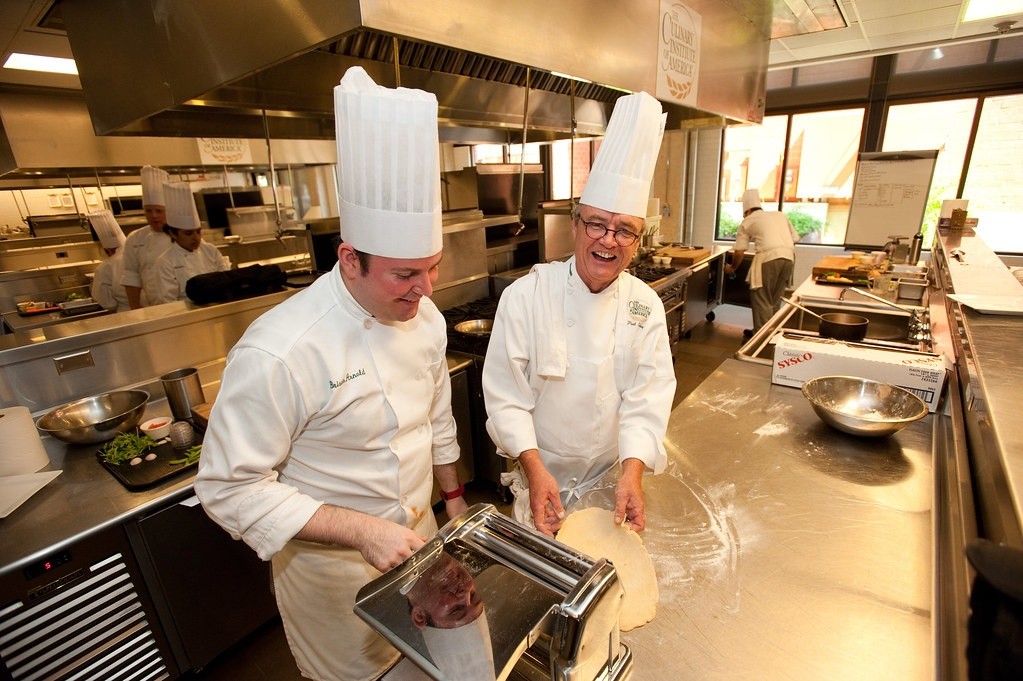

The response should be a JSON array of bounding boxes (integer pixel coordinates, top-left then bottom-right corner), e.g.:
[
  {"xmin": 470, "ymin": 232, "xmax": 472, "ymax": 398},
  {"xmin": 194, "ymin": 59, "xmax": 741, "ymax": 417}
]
[{"xmin": 778, "ymin": 293, "xmax": 870, "ymax": 342}]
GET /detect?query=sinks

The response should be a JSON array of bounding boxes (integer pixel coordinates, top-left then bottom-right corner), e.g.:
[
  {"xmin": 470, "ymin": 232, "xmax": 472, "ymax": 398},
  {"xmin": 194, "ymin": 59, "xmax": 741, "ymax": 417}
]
[
  {"xmin": 732, "ymin": 328, "xmax": 932, "ymax": 366},
  {"xmin": 781, "ymin": 295, "xmax": 925, "ymax": 347}
]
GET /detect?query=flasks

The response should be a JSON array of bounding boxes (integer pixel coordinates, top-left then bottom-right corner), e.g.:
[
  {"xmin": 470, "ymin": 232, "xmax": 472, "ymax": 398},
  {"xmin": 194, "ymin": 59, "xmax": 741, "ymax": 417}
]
[{"xmin": 906, "ymin": 233, "xmax": 923, "ymax": 266}]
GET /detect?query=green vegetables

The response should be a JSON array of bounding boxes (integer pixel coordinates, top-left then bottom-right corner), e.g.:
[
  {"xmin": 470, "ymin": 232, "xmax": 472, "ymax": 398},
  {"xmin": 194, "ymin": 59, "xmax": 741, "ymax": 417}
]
[
  {"xmin": 59, "ymin": 292, "xmax": 89, "ymax": 304},
  {"xmin": 97, "ymin": 428, "xmax": 158, "ymax": 465}
]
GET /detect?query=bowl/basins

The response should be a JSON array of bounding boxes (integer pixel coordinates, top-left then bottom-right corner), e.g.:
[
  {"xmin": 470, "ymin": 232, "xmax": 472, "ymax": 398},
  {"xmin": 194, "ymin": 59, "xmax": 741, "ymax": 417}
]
[
  {"xmin": 140, "ymin": 416, "xmax": 173, "ymax": 439},
  {"xmin": 872, "ymin": 250, "xmax": 887, "ymax": 264},
  {"xmin": 802, "ymin": 372, "xmax": 930, "ymax": 438},
  {"xmin": 33, "ymin": 301, "xmax": 45, "ymax": 309},
  {"xmin": 652, "ymin": 256, "xmax": 661, "ymax": 263},
  {"xmin": 859, "ymin": 256, "xmax": 872, "ymax": 264},
  {"xmin": 660, "ymin": 256, "xmax": 673, "ymax": 265},
  {"xmin": 851, "ymin": 251, "xmax": 866, "ymax": 259},
  {"xmin": 35, "ymin": 387, "xmax": 151, "ymax": 445}
]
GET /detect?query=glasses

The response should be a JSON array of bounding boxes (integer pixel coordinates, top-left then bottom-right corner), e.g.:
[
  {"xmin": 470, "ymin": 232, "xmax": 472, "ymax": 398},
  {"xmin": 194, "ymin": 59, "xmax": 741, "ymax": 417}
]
[{"xmin": 576, "ymin": 213, "xmax": 646, "ymax": 247}]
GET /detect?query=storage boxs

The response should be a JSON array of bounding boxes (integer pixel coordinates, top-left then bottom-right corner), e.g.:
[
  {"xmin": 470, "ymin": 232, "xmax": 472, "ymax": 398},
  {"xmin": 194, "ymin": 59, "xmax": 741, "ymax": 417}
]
[{"xmin": 769, "ymin": 331, "xmax": 955, "ymax": 413}]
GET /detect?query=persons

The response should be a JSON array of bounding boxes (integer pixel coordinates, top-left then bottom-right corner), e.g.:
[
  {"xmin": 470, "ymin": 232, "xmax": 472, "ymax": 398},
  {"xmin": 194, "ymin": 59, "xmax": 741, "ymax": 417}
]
[
  {"xmin": 482, "ymin": 93, "xmax": 676, "ymax": 538},
  {"xmin": 734, "ymin": 188, "xmax": 799, "ymax": 337},
  {"xmin": 119, "ymin": 169, "xmax": 169, "ymax": 309},
  {"xmin": 193, "ymin": 66, "xmax": 467, "ymax": 681},
  {"xmin": 88, "ymin": 209, "xmax": 147, "ymax": 312},
  {"xmin": 400, "ymin": 551, "xmax": 495, "ymax": 681},
  {"xmin": 155, "ymin": 183, "xmax": 229, "ymax": 303}
]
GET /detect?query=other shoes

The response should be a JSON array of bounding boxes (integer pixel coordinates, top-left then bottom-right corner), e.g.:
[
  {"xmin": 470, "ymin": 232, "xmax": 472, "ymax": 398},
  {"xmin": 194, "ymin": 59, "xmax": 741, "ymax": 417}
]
[{"xmin": 744, "ymin": 329, "xmax": 753, "ymax": 339}]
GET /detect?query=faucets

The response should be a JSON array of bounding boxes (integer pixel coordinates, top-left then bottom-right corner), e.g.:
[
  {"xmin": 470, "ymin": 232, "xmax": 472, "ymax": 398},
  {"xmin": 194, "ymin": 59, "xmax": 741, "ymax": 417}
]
[{"xmin": 839, "ymin": 287, "xmax": 924, "ymax": 341}]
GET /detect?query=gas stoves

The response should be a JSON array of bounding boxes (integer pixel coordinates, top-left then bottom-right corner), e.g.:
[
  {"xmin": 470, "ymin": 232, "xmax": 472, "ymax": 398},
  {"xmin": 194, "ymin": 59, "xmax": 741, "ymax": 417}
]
[{"xmin": 620, "ymin": 256, "xmax": 693, "ymax": 296}]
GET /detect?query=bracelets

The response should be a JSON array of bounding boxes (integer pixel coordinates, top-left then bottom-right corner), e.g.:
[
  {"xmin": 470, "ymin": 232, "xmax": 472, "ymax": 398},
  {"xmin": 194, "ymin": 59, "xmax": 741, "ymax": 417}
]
[{"xmin": 440, "ymin": 483, "xmax": 464, "ymax": 500}]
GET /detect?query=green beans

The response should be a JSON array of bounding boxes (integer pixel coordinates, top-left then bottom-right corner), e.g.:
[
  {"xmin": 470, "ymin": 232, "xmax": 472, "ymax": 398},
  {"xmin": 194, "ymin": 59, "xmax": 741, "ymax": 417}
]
[{"xmin": 169, "ymin": 445, "xmax": 202, "ymax": 465}]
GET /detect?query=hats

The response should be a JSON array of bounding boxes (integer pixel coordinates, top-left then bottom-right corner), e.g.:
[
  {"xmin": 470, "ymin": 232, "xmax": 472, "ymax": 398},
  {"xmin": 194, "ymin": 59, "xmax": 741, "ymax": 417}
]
[
  {"xmin": 162, "ymin": 181, "xmax": 201, "ymax": 230},
  {"xmin": 579, "ymin": 93, "xmax": 668, "ymax": 218},
  {"xmin": 334, "ymin": 66, "xmax": 442, "ymax": 260},
  {"xmin": 420, "ymin": 605, "xmax": 495, "ymax": 681},
  {"xmin": 86, "ymin": 210, "xmax": 127, "ymax": 250},
  {"xmin": 743, "ymin": 189, "xmax": 762, "ymax": 212},
  {"xmin": 141, "ymin": 165, "xmax": 169, "ymax": 207}
]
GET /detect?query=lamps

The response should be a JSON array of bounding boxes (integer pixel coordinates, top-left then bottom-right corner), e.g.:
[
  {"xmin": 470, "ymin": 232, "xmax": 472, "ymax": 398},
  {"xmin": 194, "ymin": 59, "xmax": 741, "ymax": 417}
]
[{"xmin": 931, "ymin": 48, "xmax": 944, "ymax": 60}]
